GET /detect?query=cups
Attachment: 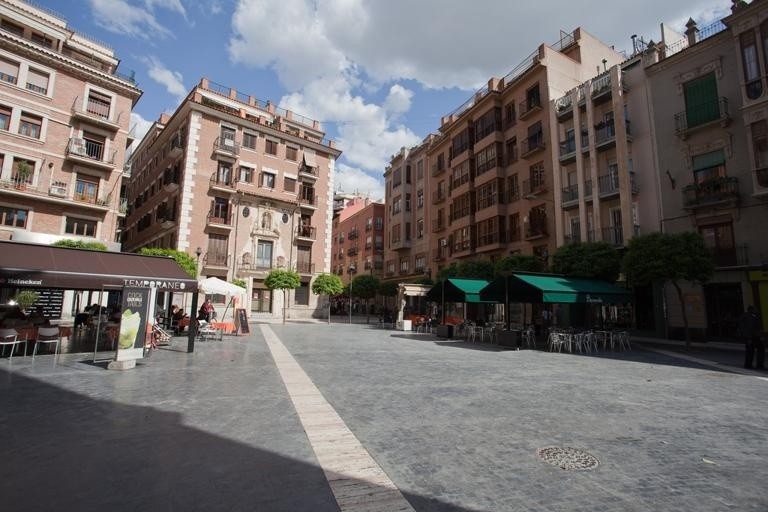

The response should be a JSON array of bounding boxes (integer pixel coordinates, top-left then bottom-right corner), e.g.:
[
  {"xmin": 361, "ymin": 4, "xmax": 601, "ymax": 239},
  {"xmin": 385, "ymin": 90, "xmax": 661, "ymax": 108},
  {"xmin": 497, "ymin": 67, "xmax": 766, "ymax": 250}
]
[{"xmin": 117, "ymin": 316, "xmax": 141, "ymax": 349}]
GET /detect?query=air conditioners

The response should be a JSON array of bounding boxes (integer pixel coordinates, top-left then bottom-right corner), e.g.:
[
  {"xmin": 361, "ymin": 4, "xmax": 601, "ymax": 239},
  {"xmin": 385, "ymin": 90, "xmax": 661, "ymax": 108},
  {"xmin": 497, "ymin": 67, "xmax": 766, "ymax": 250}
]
[
  {"xmin": 50, "ymin": 186, "xmax": 67, "ymax": 198},
  {"xmin": 69, "ymin": 144, "xmax": 86, "ymax": 155},
  {"xmin": 441, "ymin": 239, "xmax": 447, "ymax": 246},
  {"xmin": 71, "ymin": 137, "xmax": 86, "ymax": 146}
]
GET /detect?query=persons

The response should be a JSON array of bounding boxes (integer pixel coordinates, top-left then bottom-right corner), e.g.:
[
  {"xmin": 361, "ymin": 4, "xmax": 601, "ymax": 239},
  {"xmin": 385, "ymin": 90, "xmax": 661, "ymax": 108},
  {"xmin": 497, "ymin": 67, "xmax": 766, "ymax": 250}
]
[
  {"xmin": 604, "ymin": 307, "xmax": 614, "ymax": 327},
  {"xmin": 737, "ymin": 303, "xmax": 767, "ymax": 370},
  {"xmin": 172, "ymin": 309, "xmax": 188, "ymax": 333},
  {"xmin": 200, "ymin": 298, "xmax": 215, "ymax": 322}
]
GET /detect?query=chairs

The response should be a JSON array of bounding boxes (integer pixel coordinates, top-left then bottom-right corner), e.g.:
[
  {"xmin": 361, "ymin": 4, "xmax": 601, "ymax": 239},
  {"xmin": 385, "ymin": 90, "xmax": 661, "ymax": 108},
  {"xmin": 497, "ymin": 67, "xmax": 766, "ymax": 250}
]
[
  {"xmin": 405, "ymin": 313, "xmax": 632, "ymax": 355},
  {"xmin": 156, "ymin": 310, "xmax": 235, "ymax": 341},
  {"xmin": 0, "ymin": 313, "xmax": 120, "ymax": 360}
]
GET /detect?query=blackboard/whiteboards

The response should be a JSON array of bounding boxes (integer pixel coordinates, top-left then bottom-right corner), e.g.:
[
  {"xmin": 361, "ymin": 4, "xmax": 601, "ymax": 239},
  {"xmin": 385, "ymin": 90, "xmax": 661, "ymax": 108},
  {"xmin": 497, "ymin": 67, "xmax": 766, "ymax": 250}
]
[{"xmin": 239, "ymin": 310, "xmax": 249, "ymax": 334}]
